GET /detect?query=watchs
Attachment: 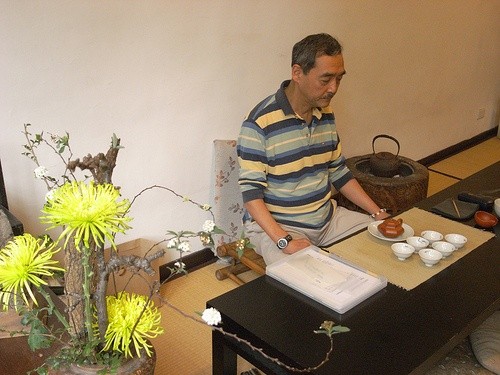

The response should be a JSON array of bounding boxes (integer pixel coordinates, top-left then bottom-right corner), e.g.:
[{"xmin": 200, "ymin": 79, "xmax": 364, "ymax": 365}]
[{"xmin": 277, "ymin": 235, "xmax": 293, "ymax": 251}]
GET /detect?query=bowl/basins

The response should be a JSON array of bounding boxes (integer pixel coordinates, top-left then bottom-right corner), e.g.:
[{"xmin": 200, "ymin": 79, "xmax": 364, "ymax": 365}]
[
  {"xmin": 432, "ymin": 242, "xmax": 455, "ymax": 256},
  {"xmin": 392, "ymin": 243, "xmax": 415, "ymax": 261},
  {"xmin": 475, "ymin": 211, "xmax": 498, "ymax": 227},
  {"xmin": 445, "ymin": 234, "xmax": 467, "ymax": 248},
  {"xmin": 419, "ymin": 249, "xmax": 442, "ymax": 265},
  {"xmin": 407, "ymin": 237, "xmax": 429, "ymax": 253},
  {"xmin": 421, "ymin": 231, "xmax": 443, "ymax": 244},
  {"xmin": 494, "ymin": 199, "xmax": 500, "ymax": 216}
]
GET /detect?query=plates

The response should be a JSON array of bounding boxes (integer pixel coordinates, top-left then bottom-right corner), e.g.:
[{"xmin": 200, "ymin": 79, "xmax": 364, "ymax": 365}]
[{"xmin": 368, "ymin": 221, "xmax": 414, "ymax": 241}]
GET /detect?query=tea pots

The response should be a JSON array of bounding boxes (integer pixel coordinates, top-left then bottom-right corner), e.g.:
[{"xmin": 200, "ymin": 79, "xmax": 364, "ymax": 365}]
[{"xmin": 378, "ymin": 218, "xmax": 404, "ymax": 237}]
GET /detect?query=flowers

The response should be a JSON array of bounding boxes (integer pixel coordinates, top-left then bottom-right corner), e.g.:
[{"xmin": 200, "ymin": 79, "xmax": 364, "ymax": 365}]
[{"xmin": 0, "ymin": 124, "xmax": 255, "ymax": 375}]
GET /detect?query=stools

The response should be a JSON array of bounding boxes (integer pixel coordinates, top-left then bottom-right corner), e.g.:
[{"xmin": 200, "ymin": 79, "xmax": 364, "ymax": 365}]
[{"xmin": 215, "ymin": 239, "xmax": 266, "ymax": 287}]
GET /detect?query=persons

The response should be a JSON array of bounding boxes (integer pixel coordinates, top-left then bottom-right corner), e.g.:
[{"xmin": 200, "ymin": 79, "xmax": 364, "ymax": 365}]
[{"xmin": 237, "ymin": 31, "xmax": 392, "ymax": 266}]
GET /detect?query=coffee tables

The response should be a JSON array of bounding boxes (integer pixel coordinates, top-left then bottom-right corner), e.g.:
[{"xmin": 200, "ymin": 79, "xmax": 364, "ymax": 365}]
[{"xmin": 198, "ymin": 159, "xmax": 500, "ymax": 375}]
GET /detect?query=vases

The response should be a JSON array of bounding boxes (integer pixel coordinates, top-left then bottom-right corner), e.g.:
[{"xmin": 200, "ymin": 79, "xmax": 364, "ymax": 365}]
[{"xmin": 65, "ymin": 328, "xmax": 156, "ymax": 375}]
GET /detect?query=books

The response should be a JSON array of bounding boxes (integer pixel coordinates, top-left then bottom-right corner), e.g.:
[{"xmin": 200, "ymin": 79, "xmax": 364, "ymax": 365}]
[{"xmin": 264, "ymin": 246, "xmax": 388, "ymax": 315}]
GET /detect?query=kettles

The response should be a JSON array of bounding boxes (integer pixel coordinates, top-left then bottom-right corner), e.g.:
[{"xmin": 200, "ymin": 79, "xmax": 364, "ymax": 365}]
[{"xmin": 369, "ymin": 135, "xmax": 402, "ymax": 177}]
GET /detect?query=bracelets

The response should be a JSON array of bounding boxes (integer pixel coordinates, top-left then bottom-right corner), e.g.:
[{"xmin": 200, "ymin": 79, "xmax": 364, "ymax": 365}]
[{"xmin": 370, "ymin": 206, "xmax": 386, "ymax": 220}]
